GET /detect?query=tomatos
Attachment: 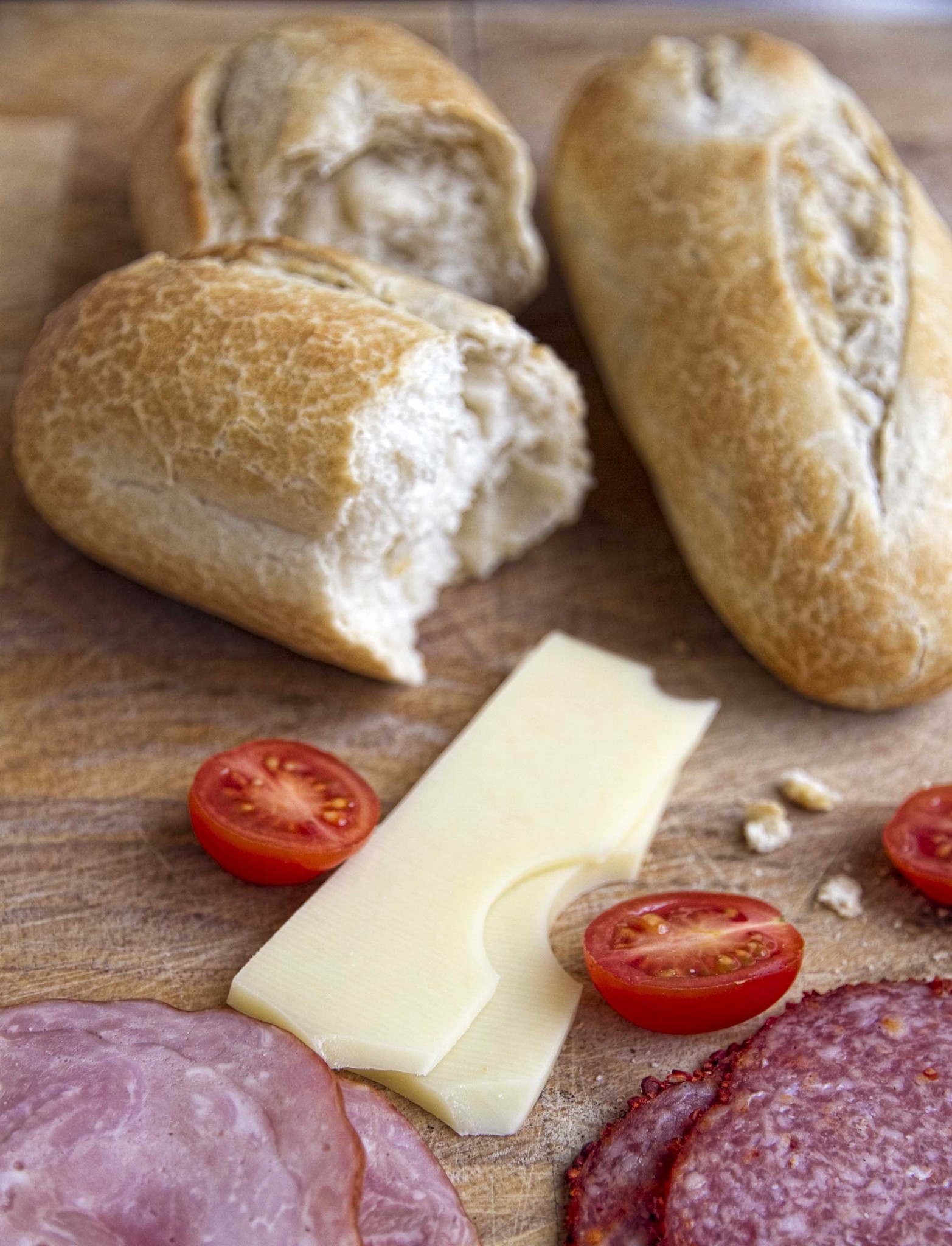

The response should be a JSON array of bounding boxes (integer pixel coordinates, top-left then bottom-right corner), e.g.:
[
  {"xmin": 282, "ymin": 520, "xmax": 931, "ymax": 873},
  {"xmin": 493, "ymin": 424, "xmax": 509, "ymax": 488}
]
[
  {"xmin": 584, "ymin": 886, "xmax": 805, "ymax": 1031},
  {"xmin": 186, "ymin": 742, "xmax": 381, "ymax": 884},
  {"xmin": 880, "ymin": 780, "xmax": 952, "ymax": 904}
]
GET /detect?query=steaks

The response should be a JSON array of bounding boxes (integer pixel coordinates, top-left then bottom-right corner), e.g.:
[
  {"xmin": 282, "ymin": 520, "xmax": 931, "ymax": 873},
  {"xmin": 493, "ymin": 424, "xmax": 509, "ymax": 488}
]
[
  {"xmin": 560, "ymin": 975, "xmax": 951, "ymax": 1246},
  {"xmin": 0, "ymin": 999, "xmax": 482, "ymax": 1246}
]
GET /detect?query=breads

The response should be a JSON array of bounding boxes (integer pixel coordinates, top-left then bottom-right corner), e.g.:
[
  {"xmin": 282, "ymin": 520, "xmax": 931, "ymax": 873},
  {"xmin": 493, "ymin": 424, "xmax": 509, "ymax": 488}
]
[
  {"xmin": 127, "ymin": 13, "xmax": 548, "ymax": 312},
  {"xmin": 547, "ymin": 31, "xmax": 952, "ymax": 714},
  {"xmin": 14, "ymin": 235, "xmax": 593, "ymax": 683}
]
